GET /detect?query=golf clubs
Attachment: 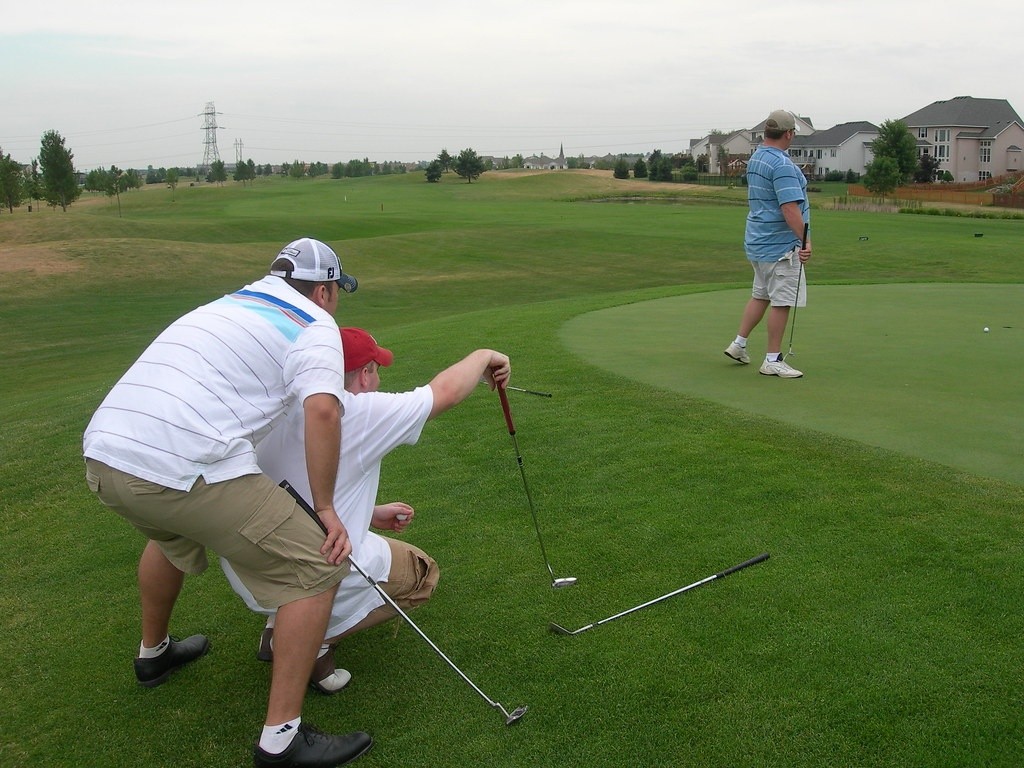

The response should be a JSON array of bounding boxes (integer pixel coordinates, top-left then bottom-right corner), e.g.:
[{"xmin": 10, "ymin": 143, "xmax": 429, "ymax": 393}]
[
  {"xmin": 277, "ymin": 479, "xmax": 531, "ymax": 726},
  {"xmin": 788, "ymin": 222, "xmax": 809, "ymax": 358},
  {"xmin": 492, "ymin": 366, "xmax": 578, "ymax": 589},
  {"xmin": 548, "ymin": 551, "xmax": 772, "ymax": 635},
  {"xmin": 480, "ymin": 380, "xmax": 552, "ymax": 398}
]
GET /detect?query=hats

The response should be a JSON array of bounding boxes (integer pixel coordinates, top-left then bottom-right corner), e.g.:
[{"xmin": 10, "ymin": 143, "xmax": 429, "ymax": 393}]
[
  {"xmin": 764, "ymin": 109, "xmax": 801, "ymax": 132},
  {"xmin": 270, "ymin": 237, "xmax": 359, "ymax": 294},
  {"xmin": 339, "ymin": 325, "xmax": 394, "ymax": 372}
]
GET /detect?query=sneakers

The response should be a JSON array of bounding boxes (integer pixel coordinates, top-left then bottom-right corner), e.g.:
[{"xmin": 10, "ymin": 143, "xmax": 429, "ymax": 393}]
[
  {"xmin": 723, "ymin": 340, "xmax": 751, "ymax": 364},
  {"xmin": 758, "ymin": 352, "xmax": 804, "ymax": 379}
]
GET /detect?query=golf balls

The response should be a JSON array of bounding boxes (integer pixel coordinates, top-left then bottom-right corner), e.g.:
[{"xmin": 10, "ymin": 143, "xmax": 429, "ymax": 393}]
[{"xmin": 983, "ymin": 327, "xmax": 989, "ymax": 333}]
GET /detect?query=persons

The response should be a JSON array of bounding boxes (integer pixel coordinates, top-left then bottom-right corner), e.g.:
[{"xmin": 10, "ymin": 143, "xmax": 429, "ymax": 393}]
[
  {"xmin": 81, "ymin": 239, "xmax": 373, "ymax": 767},
  {"xmin": 724, "ymin": 109, "xmax": 812, "ymax": 378},
  {"xmin": 220, "ymin": 327, "xmax": 512, "ymax": 694}
]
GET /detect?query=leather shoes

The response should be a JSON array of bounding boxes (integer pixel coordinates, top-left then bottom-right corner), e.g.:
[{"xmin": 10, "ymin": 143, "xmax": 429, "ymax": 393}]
[
  {"xmin": 256, "ymin": 623, "xmax": 274, "ymax": 663},
  {"xmin": 311, "ymin": 647, "xmax": 353, "ymax": 695},
  {"xmin": 252, "ymin": 723, "xmax": 375, "ymax": 768},
  {"xmin": 132, "ymin": 633, "xmax": 211, "ymax": 688}
]
